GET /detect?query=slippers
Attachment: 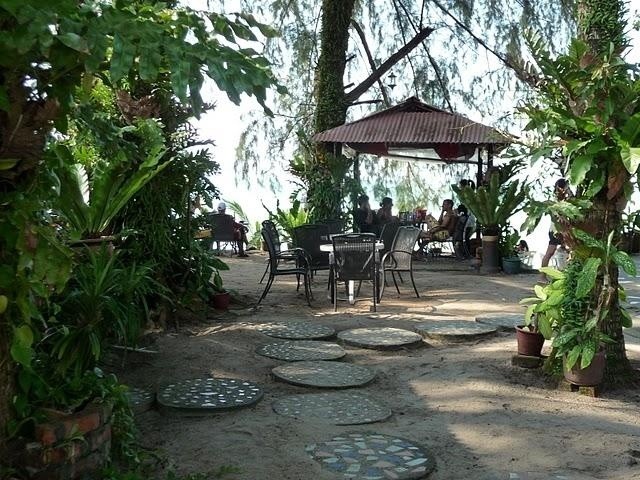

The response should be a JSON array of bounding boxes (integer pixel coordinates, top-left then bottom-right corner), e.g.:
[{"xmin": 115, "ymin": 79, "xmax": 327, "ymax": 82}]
[
  {"xmin": 245, "ymin": 245, "xmax": 258, "ymax": 251},
  {"xmin": 237, "ymin": 253, "xmax": 250, "ymax": 257}
]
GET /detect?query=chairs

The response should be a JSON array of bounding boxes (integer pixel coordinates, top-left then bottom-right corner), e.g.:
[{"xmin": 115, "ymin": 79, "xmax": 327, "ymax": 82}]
[
  {"xmin": 257, "ymin": 206, "xmax": 469, "ymax": 314},
  {"xmin": 208, "ymin": 211, "xmax": 244, "ymax": 260}
]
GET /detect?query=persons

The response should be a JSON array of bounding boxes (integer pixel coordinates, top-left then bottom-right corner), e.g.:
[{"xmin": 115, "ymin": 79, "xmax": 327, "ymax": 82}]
[
  {"xmin": 377, "ymin": 197, "xmax": 393, "ymax": 218},
  {"xmin": 416, "ymin": 199, "xmax": 457, "ymax": 257},
  {"xmin": 459, "ymin": 179, "xmax": 475, "ymax": 257},
  {"xmin": 356, "ymin": 195, "xmax": 398, "ymax": 237},
  {"xmin": 217, "ymin": 202, "xmax": 258, "ymax": 257},
  {"xmin": 542, "ymin": 179, "xmax": 573, "ymax": 267},
  {"xmin": 517, "ymin": 240, "xmax": 528, "ymax": 252}
]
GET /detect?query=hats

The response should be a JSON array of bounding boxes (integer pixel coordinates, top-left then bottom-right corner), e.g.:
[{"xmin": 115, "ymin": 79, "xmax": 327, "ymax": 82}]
[{"xmin": 216, "ymin": 202, "xmax": 227, "ymax": 211}]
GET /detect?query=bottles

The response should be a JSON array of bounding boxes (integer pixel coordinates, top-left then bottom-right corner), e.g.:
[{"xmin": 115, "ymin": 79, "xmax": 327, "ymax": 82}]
[{"xmin": 405, "ymin": 212, "xmax": 417, "ymax": 222}]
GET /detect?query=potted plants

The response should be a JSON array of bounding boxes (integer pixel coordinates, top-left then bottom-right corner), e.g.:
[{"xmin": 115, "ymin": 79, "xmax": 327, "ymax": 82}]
[
  {"xmin": 499, "ymin": 227, "xmax": 529, "ymax": 273},
  {"xmin": 535, "ymin": 227, "xmax": 633, "ymax": 387},
  {"xmin": 454, "ymin": 168, "xmax": 535, "ymax": 275},
  {"xmin": 514, "ymin": 284, "xmax": 550, "ymax": 357}
]
